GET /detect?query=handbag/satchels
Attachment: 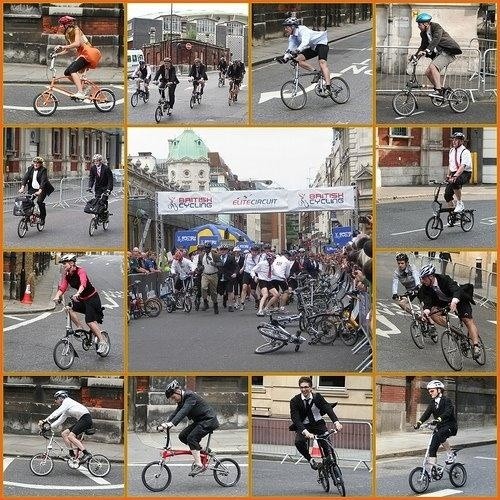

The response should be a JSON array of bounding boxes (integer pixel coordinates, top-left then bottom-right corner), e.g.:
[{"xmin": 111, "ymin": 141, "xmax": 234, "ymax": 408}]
[{"xmin": 84, "ymin": 197, "xmax": 106, "ymax": 214}]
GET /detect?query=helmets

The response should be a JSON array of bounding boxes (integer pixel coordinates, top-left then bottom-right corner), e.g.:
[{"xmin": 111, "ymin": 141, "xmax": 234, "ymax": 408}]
[
  {"xmin": 419, "ymin": 264, "xmax": 436, "ymax": 278},
  {"xmin": 427, "ymin": 380, "xmax": 444, "ymax": 390},
  {"xmin": 92, "ymin": 153, "xmax": 103, "ymax": 161},
  {"xmin": 58, "ymin": 253, "xmax": 77, "ymax": 262},
  {"xmin": 450, "ymin": 131, "xmax": 466, "ymax": 139},
  {"xmin": 396, "ymin": 253, "xmax": 409, "ymax": 262},
  {"xmin": 416, "ymin": 14, "xmax": 432, "ymax": 22},
  {"xmin": 32, "ymin": 157, "xmax": 45, "ymax": 163},
  {"xmin": 166, "ymin": 378, "xmax": 180, "ymax": 399},
  {"xmin": 54, "ymin": 390, "xmax": 69, "ymax": 399},
  {"xmin": 282, "ymin": 16, "xmax": 299, "ymax": 25},
  {"xmin": 281, "ymin": 248, "xmax": 305, "ymax": 256},
  {"xmin": 174, "ymin": 243, "xmax": 271, "ymax": 261},
  {"xmin": 58, "ymin": 16, "xmax": 76, "ymax": 24}
]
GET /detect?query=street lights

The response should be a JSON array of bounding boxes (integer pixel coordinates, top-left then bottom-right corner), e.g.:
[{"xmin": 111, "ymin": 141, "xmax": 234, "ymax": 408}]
[{"xmin": 326, "ymin": 156, "xmax": 342, "ymax": 187}]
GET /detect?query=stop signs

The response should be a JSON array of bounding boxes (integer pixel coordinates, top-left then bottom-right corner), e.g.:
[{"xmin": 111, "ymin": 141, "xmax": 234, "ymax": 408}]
[{"xmin": 186, "ymin": 43, "xmax": 192, "ymax": 51}]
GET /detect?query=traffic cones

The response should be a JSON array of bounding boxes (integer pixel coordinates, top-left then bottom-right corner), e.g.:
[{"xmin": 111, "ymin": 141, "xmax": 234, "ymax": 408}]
[
  {"xmin": 310, "ymin": 438, "xmax": 321, "ymax": 457},
  {"xmin": 20, "ymin": 282, "xmax": 33, "ymax": 305}
]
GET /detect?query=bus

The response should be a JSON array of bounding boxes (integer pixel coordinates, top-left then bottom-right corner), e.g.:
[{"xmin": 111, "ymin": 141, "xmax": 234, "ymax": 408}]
[{"xmin": 127, "ymin": 49, "xmax": 144, "ymax": 79}]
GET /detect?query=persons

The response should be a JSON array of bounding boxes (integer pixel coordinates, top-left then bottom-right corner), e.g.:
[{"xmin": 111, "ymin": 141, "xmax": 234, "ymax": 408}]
[
  {"xmin": 217, "ymin": 57, "xmax": 228, "ymax": 83},
  {"xmin": 444, "ymin": 131, "xmax": 474, "ymax": 228},
  {"xmin": 131, "ymin": 59, "xmax": 151, "ymax": 94},
  {"xmin": 416, "ymin": 263, "xmax": 481, "ymax": 357},
  {"xmin": 415, "ymin": 11, "xmax": 464, "ymax": 99},
  {"xmin": 86, "ymin": 152, "xmax": 116, "ymax": 225},
  {"xmin": 188, "ymin": 58, "xmax": 208, "ymax": 93},
  {"xmin": 53, "ymin": 16, "xmax": 102, "ymax": 100},
  {"xmin": 159, "ymin": 380, "xmax": 221, "ymax": 477},
  {"xmin": 127, "ymin": 214, "xmax": 372, "ymax": 319},
  {"xmin": 412, "ymin": 379, "xmax": 459, "ymax": 466},
  {"xmin": 37, "ymin": 389, "xmax": 95, "ymax": 464},
  {"xmin": 277, "ymin": 16, "xmax": 333, "ymax": 97},
  {"xmin": 13, "ymin": 155, "xmax": 55, "ymax": 228},
  {"xmin": 389, "ymin": 253, "xmax": 435, "ymax": 326},
  {"xmin": 228, "ymin": 58, "xmax": 245, "ymax": 96},
  {"xmin": 152, "ymin": 56, "xmax": 179, "ymax": 115},
  {"xmin": 52, "ymin": 255, "xmax": 109, "ymax": 356},
  {"xmin": 290, "ymin": 376, "xmax": 344, "ymax": 470}
]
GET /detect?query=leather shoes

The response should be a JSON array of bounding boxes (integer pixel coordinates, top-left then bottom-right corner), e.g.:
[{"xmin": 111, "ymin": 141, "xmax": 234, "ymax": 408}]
[
  {"xmin": 309, "ymin": 460, "xmax": 318, "ymax": 470},
  {"xmin": 40, "ymin": 219, "xmax": 45, "ymax": 225}
]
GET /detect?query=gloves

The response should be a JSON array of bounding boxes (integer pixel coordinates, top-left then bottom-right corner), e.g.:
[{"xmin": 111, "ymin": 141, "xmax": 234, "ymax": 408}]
[{"xmin": 393, "ymin": 294, "xmax": 399, "ymax": 300}]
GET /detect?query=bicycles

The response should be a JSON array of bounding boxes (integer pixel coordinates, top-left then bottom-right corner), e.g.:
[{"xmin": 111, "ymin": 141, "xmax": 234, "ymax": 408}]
[
  {"xmin": 425, "ymin": 179, "xmax": 476, "ymax": 241},
  {"xmin": 129, "ymin": 272, "xmax": 200, "ymax": 321},
  {"xmin": 17, "ymin": 192, "xmax": 44, "ymax": 238},
  {"xmin": 130, "ymin": 77, "xmax": 149, "ymax": 108},
  {"xmin": 227, "ymin": 77, "xmax": 238, "ymax": 106},
  {"xmin": 29, "ymin": 420, "xmax": 111, "ymax": 478},
  {"xmin": 302, "ymin": 428, "xmax": 346, "ymax": 498},
  {"xmin": 396, "ymin": 290, "xmax": 438, "ymax": 349},
  {"xmin": 428, "ymin": 306, "xmax": 487, "ymax": 372},
  {"xmin": 53, "ymin": 295, "xmax": 112, "ymax": 370},
  {"xmin": 155, "ymin": 82, "xmax": 176, "ymax": 123},
  {"xmin": 391, "ymin": 52, "xmax": 471, "ymax": 118},
  {"xmin": 253, "ymin": 270, "xmax": 359, "ymax": 354},
  {"xmin": 280, "ymin": 51, "xmax": 351, "ymax": 110},
  {"xmin": 408, "ymin": 418, "xmax": 467, "ymax": 494},
  {"xmin": 217, "ymin": 69, "xmax": 225, "ymax": 88},
  {"xmin": 86, "ymin": 190, "xmax": 113, "ymax": 236},
  {"xmin": 142, "ymin": 423, "xmax": 241, "ymax": 492},
  {"xmin": 32, "ymin": 46, "xmax": 115, "ymax": 116},
  {"xmin": 189, "ymin": 80, "xmax": 201, "ymax": 107}
]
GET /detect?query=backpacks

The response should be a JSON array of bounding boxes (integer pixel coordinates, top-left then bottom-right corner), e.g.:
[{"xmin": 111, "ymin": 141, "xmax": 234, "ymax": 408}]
[{"xmin": 13, "ymin": 195, "xmax": 35, "ymax": 216}]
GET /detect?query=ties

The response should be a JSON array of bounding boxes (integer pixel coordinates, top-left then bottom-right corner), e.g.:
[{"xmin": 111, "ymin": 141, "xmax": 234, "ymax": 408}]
[
  {"xmin": 455, "ymin": 148, "xmax": 460, "ymax": 169},
  {"xmin": 221, "ymin": 255, "xmax": 225, "ymax": 263},
  {"xmin": 268, "ymin": 265, "xmax": 271, "ymax": 278},
  {"xmin": 304, "ymin": 399, "xmax": 316, "ymax": 423},
  {"xmin": 206, "ymin": 253, "xmax": 212, "ymax": 264},
  {"xmin": 252, "ymin": 257, "xmax": 256, "ymax": 266}
]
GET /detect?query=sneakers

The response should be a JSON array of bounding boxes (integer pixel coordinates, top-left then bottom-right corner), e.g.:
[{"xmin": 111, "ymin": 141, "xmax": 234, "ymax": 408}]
[
  {"xmin": 454, "ymin": 202, "xmax": 464, "ymax": 213},
  {"xmin": 79, "ymin": 453, "xmax": 92, "ymax": 464},
  {"xmin": 70, "ymin": 92, "xmax": 85, "ymax": 100},
  {"xmin": 188, "ymin": 464, "xmax": 205, "ymax": 476},
  {"xmin": 472, "ymin": 344, "xmax": 482, "ymax": 359},
  {"xmin": 428, "ymin": 90, "xmax": 445, "ymax": 99},
  {"xmin": 96, "ymin": 342, "xmax": 108, "ymax": 353},
  {"xmin": 312, "ymin": 74, "xmax": 320, "ymax": 82},
  {"xmin": 168, "ymin": 107, "xmax": 173, "ymax": 114},
  {"xmin": 202, "ymin": 298, "xmax": 265, "ymax": 316},
  {"xmin": 320, "ymin": 89, "xmax": 332, "ymax": 97},
  {"xmin": 445, "ymin": 454, "xmax": 457, "ymax": 463}
]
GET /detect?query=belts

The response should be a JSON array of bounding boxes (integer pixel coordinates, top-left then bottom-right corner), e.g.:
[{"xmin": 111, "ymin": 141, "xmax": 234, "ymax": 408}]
[{"xmin": 207, "ymin": 272, "xmax": 218, "ymax": 276}]
